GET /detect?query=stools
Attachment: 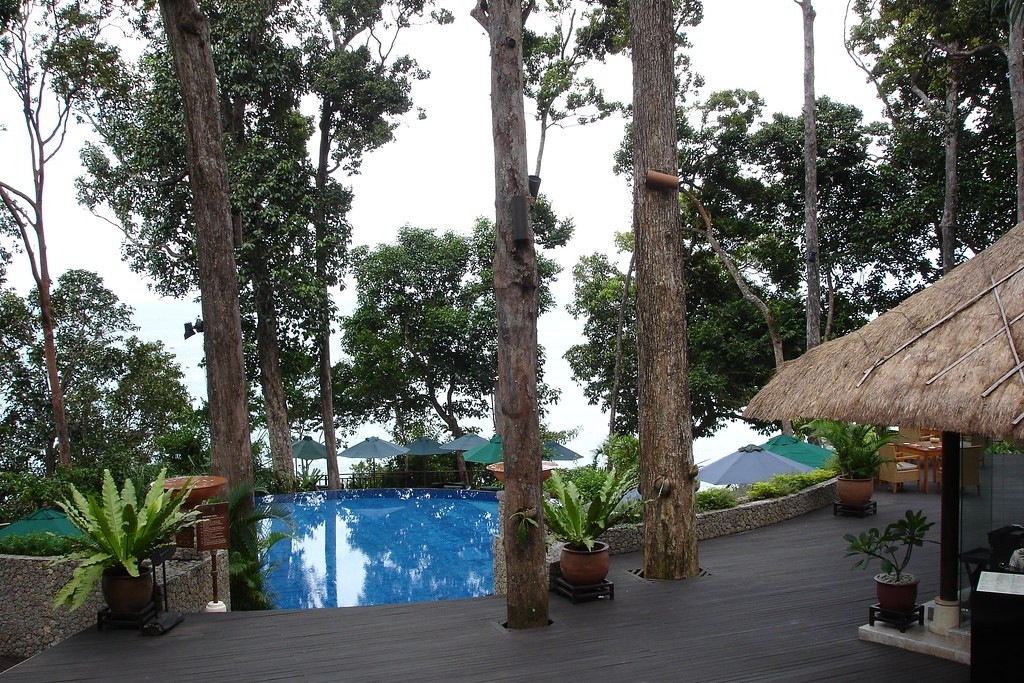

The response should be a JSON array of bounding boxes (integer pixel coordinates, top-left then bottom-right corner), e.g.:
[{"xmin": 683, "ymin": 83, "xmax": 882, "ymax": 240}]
[{"xmin": 958, "ymin": 546, "xmax": 994, "ymax": 610}]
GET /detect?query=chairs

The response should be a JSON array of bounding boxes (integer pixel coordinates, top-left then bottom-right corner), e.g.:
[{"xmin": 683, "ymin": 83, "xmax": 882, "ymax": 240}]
[
  {"xmin": 878, "ymin": 445, "xmax": 921, "ymax": 495},
  {"xmin": 960, "ymin": 444, "xmax": 983, "ymax": 497}
]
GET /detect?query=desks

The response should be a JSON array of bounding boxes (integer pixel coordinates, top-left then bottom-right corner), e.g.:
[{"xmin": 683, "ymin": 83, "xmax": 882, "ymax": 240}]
[
  {"xmin": 897, "ymin": 441, "xmax": 943, "ymax": 494},
  {"xmin": 968, "ymin": 569, "xmax": 1024, "ymax": 683}
]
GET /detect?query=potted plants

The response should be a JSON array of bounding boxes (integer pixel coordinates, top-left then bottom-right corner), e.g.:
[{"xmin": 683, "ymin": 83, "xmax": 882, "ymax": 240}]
[
  {"xmin": 540, "ymin": 465, "xmax": 641, "ymax": 587},
  {"xmin": 45, "ymin": 464, "xmax": 207, "ymax": 614},
  {"xmin": 842, "ymin": 508, "xmax": 941, "ymax": 611},
  {"xmin": 799, "ymin": 418, "xmax": 904, "ymax": 507}
]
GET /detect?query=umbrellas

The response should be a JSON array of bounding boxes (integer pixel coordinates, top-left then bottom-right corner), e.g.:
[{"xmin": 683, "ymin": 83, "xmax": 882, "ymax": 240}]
[
  {"xmin": 264, "ymin": 436, "xmax": 338, "ymax": 480},
  {"xmin": 0, "ymin": 503, "xmax": 89, "ymax": 540},
  {"xmin": 695, "ymin": 434, "xmax": 841, "ymax": 485},
  {"xmin": 338, "ymin": 432, "xmax": 584, "ymax": 488}
]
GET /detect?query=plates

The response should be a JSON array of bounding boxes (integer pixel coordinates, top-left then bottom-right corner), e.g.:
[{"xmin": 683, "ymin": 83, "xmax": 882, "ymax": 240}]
[{"xmin": 929, "ymin": 447, "xmax": 936, "ymax": 449}]
